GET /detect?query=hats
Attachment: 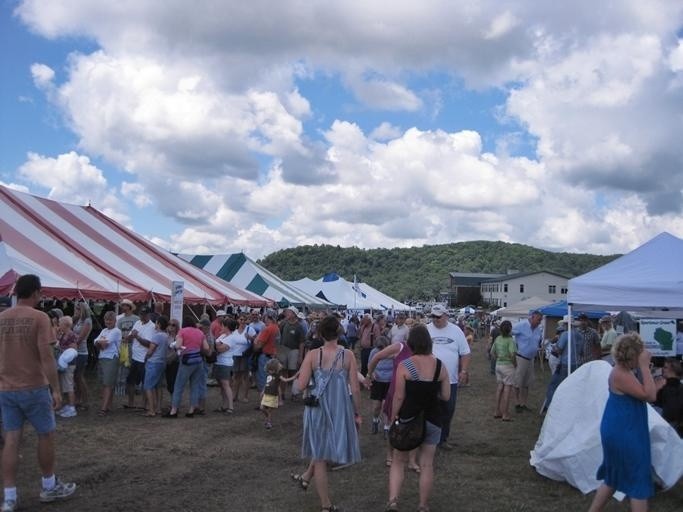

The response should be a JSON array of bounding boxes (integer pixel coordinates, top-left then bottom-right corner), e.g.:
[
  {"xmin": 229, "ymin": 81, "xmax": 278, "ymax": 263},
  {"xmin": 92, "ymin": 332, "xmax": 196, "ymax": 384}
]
[
  {"xmin": 429, "ymin": 305, "xmax": 448, "ymax": 317},
  {"xmin": 297, "ymin": 312, "xmax": 305, "ymax": 318},
  {"xmin": 288, "ymin": 306, "xmax": 298, "ymax": 315},
  {"xmin": 216, "ymin": 310, "xmax": 229, "ymax": 317},
  {"xmin": 558, "ymin": 315, "xmax": 580, "ymax": 327},
  {"xmin": 120, "ymin": 299, "xmax": 136, "ymax": 311}
]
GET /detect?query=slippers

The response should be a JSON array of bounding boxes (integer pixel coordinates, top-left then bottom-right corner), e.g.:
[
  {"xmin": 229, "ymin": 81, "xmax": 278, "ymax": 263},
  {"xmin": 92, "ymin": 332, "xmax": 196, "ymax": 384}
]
[
  {"xmin": 494, "ymin": 415, "xmax": 502, "ymax": 419},
  {"xmin": 502, "ymin": 416, "xmax": 516, "ymax": 422}
]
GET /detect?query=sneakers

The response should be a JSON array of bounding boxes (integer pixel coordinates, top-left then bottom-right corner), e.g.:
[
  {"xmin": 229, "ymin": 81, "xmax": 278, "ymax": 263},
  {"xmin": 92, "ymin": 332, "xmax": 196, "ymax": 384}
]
[
  {"xmin": 383, "ymin": 424, "xmax": 391, "ymax": 440},
  {"xmin": 372, "ymin": 416, "xmax": 381, "ymax": 434},
  {"xmin": 1, "ymin": 495, "xmax": 18, "ymax": 511},
  {"xmin": 56, "ymin": 404, "xmax": 69, "ymax": 415},
  {"xmin": 515, "ymin": 404, "xmax": 523, "ymax": 414},
  {"xmin": 39, "ymin": 480, "xmax": 77, "ymax": 502},
  {"xmin": 439, "ymin": 440, "xmax": 452, "ymax": 450},
  {"xmin": 522, "ymin": 405, "xmax": 532, "ymax": 412},
  {"xmin": 60, "ymin": 408, "xmax": 77, "ymax": 418}
]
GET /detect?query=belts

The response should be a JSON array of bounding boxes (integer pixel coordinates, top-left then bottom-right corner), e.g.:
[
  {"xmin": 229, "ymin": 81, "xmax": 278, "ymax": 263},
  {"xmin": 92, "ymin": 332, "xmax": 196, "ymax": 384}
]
[{"xmin": 516, "ymin": 353, "xmax": 532, "ymax": 360}]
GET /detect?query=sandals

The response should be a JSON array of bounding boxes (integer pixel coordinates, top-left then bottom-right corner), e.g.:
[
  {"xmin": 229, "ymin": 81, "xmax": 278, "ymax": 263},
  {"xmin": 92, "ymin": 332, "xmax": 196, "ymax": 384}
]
[
  {"xmin": 290, "ymin": 459, "xmax": 430, "ymax": 512},
  {"xmin": 97, "ymin": 395, "xmax": 299, "ymax": 430}
]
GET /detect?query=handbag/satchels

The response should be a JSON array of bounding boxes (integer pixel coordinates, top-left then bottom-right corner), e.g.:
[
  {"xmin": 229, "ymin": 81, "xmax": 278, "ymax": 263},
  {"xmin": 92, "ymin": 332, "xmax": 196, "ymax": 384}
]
[
  {"xmin": 56, "ymin": 352, "xmax": 65, "ymax": 372},
  {"xmin": 242, "ymin": 346, "xmax": 257, "ymax": 371},
  {"xmin": 206, "ymin": 352, "xmax": 217, "ymax": 362},
  {"xmin": 388, "ymin": 410, "xmax": 427, "ymax": 452}
]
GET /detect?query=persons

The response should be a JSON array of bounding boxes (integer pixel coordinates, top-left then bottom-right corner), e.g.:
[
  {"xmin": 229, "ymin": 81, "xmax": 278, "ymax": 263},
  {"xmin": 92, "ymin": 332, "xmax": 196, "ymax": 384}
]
[
  {"xmin": 288, "ymin": 315, "xmax": 363, "ymax": 512},
  {"xmin": 1, "ymin": 274, "xmax": 77, "ymax": 512},
  {"xmin": 489, "ymin": 310, "xmax": 683, "ymax": 439},
  {"xmin": 46, "ymin": 298, "xmax": 488, "ymax": 472},
  {"xmin": 382, "ymin": 324, "xmax": 451, "ymax": 511},
  {"xmin": 588, "ymin": 329, "xmax": 666, "ymax": 512}
]
